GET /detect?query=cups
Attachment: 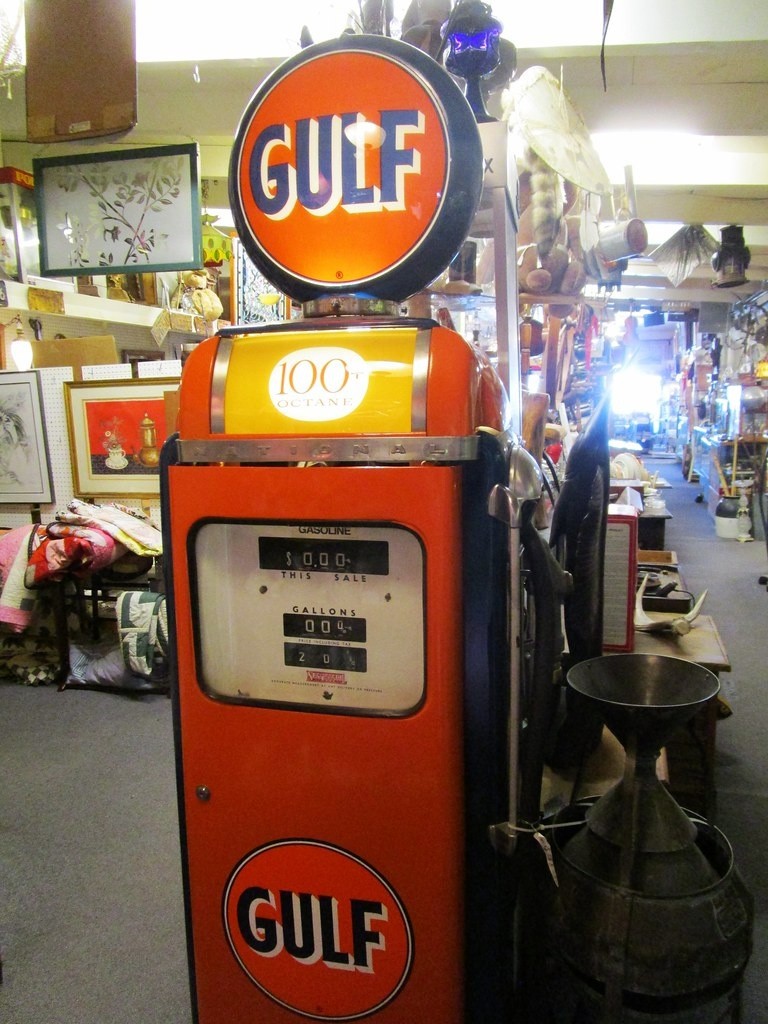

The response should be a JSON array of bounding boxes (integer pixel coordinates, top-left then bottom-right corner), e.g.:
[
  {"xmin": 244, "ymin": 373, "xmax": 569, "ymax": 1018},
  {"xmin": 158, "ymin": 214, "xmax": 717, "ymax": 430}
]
[{"xmin": 109, "ymin": 448, "xmax": 126, "ymax": 462}]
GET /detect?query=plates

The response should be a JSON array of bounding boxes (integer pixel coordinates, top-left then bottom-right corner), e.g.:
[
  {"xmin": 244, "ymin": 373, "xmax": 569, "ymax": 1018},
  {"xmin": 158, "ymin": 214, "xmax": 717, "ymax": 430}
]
[{"xmin": 105, "ymin": 457, "xmax": 128, "ymax": 469}]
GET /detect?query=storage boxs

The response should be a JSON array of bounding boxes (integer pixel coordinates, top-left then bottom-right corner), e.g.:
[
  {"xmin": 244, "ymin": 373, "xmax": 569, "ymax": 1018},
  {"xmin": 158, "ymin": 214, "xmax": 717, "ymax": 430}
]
[{"xmin": 601, "ymin": 503, "xmax": 637, "ymax": 654}]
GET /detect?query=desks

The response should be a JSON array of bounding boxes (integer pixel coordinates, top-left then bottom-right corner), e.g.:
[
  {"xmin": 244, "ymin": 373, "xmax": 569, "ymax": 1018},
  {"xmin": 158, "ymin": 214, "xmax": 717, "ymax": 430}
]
[{"xmin": 605, "ymin": 612, "xmax": 731, "ymax": 827}]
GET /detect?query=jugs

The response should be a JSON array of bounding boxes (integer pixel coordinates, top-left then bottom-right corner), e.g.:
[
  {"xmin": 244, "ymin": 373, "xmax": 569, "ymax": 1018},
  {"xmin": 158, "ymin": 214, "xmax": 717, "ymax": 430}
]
[{"xmin": 130, "ymin": 413, "xmax": 162, "ymax": 467}]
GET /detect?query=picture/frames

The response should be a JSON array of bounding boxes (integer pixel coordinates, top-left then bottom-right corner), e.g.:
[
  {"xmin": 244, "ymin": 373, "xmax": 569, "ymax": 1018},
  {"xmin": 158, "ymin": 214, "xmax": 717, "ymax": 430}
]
[
  {"xmin": 228, "ymin": 230, "xmax": 291, "ymax": 327},
  {"xmin": 63, "ymin": 376, "xmax": 182, "ymax": 499},
  {"xmin": 0, "ymin": 370, "xmax": 57, "ymax": 504},
  {"xmin": 32, "ymin": 141, "xmax": 205, "ymax": 278}
]
